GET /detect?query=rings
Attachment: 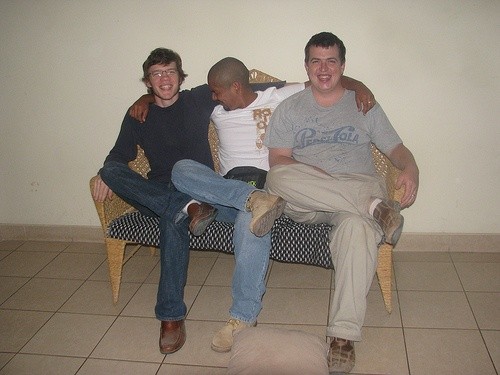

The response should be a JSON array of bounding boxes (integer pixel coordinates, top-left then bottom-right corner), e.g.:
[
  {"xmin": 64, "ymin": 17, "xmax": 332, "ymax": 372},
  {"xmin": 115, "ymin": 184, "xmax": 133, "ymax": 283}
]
[
  {"xmin": 368, "ymin": 101, "xmax": 370, "ymax": 103},
  {"xmin": 412, "ymin": 195, "xmax": 415, "ymax": 196}
]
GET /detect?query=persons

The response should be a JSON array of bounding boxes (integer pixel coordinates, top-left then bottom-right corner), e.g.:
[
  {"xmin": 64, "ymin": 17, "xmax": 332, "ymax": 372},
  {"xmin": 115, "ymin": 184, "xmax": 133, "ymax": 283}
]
[
  {"xmin": 262, "ymin": 32, "xmax": 419, "ymax": 375},
  {"xmin": 129, "ymin": 57, "xmax": 376, "ymax": 352},
  {"xmin": 92, "ymin": 47, "xmax": 300, "ymax": 354}
]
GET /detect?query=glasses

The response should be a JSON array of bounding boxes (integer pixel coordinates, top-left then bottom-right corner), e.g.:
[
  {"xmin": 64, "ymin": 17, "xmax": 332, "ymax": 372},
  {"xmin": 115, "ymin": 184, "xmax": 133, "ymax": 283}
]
[{"xmin": 147, "ymin": 70, "xmax": 179, "ymax": 77}]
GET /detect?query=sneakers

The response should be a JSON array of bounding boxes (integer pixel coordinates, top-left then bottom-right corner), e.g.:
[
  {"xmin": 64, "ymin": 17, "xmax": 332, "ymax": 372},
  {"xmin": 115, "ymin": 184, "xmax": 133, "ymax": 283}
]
[
  {"xmin": 246, "ymin": 190, "xmax": 283, "ymax": 237},
  {"xmin": 211, "ymin": 317, "xmax": 257, "ymax": 353}
]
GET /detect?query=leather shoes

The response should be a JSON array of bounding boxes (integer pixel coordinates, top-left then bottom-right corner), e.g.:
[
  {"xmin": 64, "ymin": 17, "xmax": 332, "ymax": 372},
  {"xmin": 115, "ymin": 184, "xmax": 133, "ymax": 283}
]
[
  {"xmin": 327, "ymin": 337, "xmax": 355, "ymax": 375},
  {"xmin": 159, "ymin": 320, "xmax": 186, "ymax": 354},
  {"xmin": 187, "ymin": 202, "xmax": 219, "ymax": 236},
  {"xmin": 373, "ymin": 200, "xmax": 404, "ymax": 244}
]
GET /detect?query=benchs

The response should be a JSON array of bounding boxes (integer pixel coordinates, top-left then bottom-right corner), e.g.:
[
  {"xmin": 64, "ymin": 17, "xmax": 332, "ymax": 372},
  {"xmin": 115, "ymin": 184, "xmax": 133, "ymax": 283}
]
[{"xmin": 88, "ymin": 68, "xmax": 412, "ymax": 315}]
